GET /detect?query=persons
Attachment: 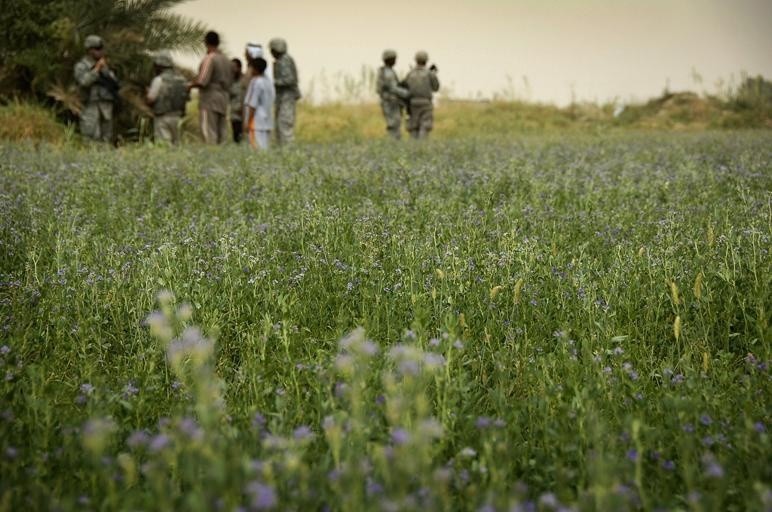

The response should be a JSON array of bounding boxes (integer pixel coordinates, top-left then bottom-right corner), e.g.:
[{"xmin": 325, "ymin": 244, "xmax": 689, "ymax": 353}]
[
  {"xmin": 74, "ymin": 32, "xmax": 120, "ymax": 148},
  {"xmin": 185, "ymin": 30, "xmax": 302, "ymax": 146},
  {"xmin": 146, "ymin": 54, "xmax": 191, "ymax": 149},
  {"xmin": 376, "ymin": 49, "xmax": 410, "ymax": 141},
  {"xmin": 402, "ymin": 51, "xmax": 439, "ymax": 140}
]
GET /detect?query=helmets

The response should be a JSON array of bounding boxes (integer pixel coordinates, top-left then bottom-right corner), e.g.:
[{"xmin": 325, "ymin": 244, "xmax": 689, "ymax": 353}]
[
  {"xmin": 83, "ymin": 34, "xmax": 103, "ymax": 51},
  {"xmin": 269, "ymin": 38, "xmax": 286, "ymax": 53},
  {"xmin": 382, "ymin": 50, "xmax": 397, "ymax": 60},
  {"xmin": 415, "ymin": 51, "xmax": 428, "ymax": 65},
  {"xmin": 155, "ymin": 54, "xmax": 172, "ymax": 67}
]
